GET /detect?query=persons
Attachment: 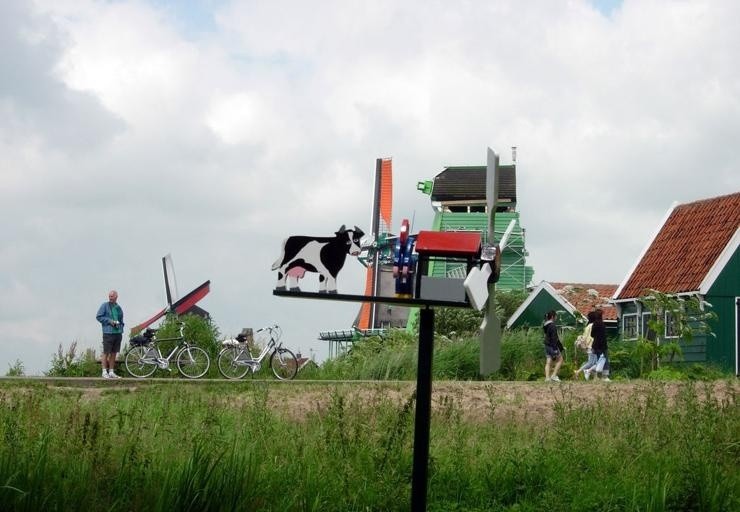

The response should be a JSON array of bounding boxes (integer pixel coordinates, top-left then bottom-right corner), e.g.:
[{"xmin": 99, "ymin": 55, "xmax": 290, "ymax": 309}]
[
  {"xmin": 543, "ymin": 310, "xmax": 564, "ymax": 382},
  {"xmin": 96, "ymin": 290, "xmax": 125, "ymax": 379},
  {"xmin": 572, "ymin": 311, "xmax": 598, "ymax": 381},
  {"xmin": 583, "ymin": 309, "xmax": 614, "ymax": 381}
]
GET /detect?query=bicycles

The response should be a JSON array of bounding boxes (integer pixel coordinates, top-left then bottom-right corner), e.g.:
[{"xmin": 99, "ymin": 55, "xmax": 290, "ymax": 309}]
[
  {"xmin": 125, "ymin": 321, "xmax": 211, "ymax": 379},
  {"xmin": 216, "ymin": 324, "xmax": 299, "ymax": 380}
]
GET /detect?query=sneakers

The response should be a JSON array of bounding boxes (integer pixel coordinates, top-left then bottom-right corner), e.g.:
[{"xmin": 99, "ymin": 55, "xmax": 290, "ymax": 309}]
[
  {"xmin": 583, "ymin": 369, "xmax": 591, "ymax": 380},
  {"xmin": 102, "ymin": 373, "xmax": 110, "ymax": 378},
  {"xmin": 108, "ymin": 373, "xmax": 119, "ymax": 378},
  {"xmin": 574, "ymin": 370, "xmax": 579, "ymax": 380},
  {"xmin": 601, "ymin": 377, "xmax": 612, "ymax": 382},
  {"xmin": 545, "ymin": 375, "xmax": 559, "ymax": 381}
]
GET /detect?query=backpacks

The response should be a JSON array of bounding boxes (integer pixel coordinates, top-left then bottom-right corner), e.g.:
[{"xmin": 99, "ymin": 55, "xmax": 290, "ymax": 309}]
[{"xmin": 575, "ymin": 335, "xmax": 587, "ymax": 349}]
